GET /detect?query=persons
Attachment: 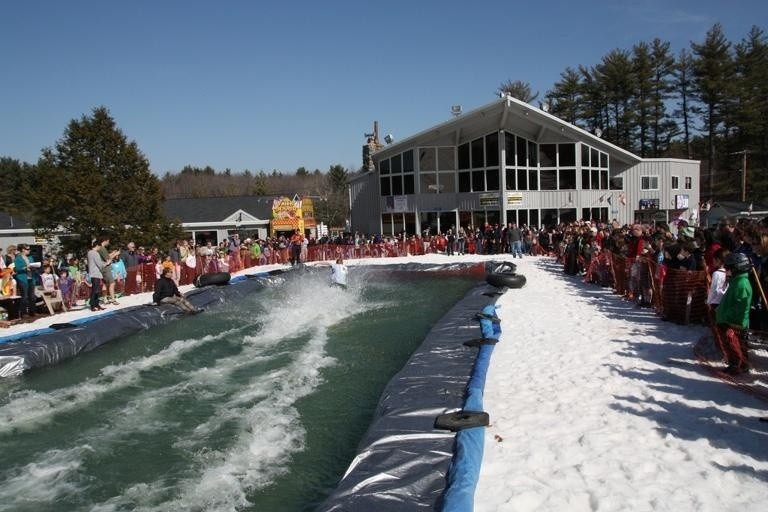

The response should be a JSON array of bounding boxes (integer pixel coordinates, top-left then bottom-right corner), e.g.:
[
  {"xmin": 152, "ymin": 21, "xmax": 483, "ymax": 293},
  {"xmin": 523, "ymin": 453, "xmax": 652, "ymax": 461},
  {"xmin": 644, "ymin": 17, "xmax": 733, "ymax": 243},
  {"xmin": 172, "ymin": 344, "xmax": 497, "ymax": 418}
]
[{"xmin": 0, "ymin": 220, "xmax": 768, "ymax": 377}]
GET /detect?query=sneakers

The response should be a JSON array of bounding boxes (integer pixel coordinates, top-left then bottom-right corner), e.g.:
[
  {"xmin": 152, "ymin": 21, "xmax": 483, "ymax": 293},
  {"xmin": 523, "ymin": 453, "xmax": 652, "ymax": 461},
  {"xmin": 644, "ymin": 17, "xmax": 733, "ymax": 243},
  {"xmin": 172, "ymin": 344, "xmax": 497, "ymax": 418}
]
[{"xmin": 722, "ymin": 366, "xmax": 749, "ymax": 376}]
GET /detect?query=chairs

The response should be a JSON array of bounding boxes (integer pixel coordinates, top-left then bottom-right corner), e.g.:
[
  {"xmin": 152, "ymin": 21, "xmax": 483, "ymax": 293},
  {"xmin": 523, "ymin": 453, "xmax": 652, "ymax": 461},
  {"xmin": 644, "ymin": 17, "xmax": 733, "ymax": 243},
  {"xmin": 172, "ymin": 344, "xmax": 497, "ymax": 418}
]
[{"xmin": 32, "ymin": 286, "xmax": 66, "ymax": 317}]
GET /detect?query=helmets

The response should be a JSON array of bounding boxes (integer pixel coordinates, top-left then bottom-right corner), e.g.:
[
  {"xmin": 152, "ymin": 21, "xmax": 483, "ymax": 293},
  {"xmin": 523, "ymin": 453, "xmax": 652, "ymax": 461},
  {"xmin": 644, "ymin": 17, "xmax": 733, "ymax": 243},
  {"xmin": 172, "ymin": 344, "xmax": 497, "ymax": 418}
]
[{"xmin": 723, "ymin": 252, "xmax": 752, "ymax": 274}]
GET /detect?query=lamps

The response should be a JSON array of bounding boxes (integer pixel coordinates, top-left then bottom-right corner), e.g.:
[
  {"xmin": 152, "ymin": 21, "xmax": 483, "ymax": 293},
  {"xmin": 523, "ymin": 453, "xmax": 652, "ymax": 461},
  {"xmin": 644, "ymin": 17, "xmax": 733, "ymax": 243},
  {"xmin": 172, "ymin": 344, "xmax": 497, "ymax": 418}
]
[
  {"xmin": 538, "ymin": 98, "xmax": 548, "ymax": 113},
  {"xmin": 449, "ymin": 104, "xmax": 463, "ymax": 117},
  {"xmin": 594, "ymin": 127, "xmax": 603, "ymax": 139},
  {"xmin": 383, "ymin": 134, "xmax": 394, "ymax": 143}
]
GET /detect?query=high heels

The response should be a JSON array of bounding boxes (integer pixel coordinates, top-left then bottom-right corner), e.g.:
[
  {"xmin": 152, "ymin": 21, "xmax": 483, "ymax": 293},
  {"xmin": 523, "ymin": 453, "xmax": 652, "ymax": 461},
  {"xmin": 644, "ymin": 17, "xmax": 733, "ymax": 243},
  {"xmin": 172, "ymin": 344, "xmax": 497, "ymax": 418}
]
[{"xmin": 103, "ymin": 299, "xmax": 120, "ymax": 306}]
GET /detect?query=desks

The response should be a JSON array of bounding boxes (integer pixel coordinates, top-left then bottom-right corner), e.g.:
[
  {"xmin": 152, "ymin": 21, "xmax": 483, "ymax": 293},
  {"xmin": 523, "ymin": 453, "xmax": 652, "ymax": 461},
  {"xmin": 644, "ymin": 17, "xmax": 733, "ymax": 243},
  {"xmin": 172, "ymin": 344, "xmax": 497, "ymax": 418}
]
[{"xmin": 1, "ymin": 294, "xmax": 23, "ymax": 323}]
